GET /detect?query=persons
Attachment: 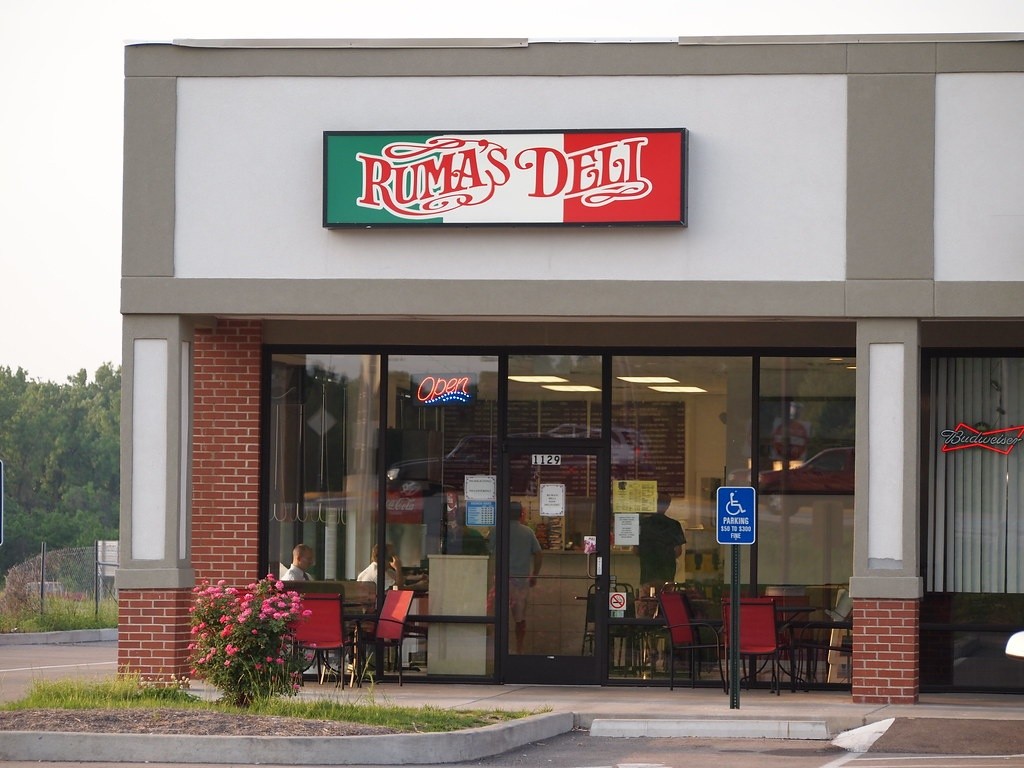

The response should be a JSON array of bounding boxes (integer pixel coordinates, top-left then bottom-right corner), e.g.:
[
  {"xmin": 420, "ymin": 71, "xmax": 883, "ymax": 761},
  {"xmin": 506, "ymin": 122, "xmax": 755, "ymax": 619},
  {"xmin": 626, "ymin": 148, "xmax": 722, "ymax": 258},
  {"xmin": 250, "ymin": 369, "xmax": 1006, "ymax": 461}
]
[
  {"xmin": 632, "ymin": 492, "xmax": 688, "ymax": 665},
  {"xmin": 280, "ymin": 541, "xmax": 361, "ymax": 672},
  {"xmin": 485, "ymin": 500, "xmax": 543, "ymax": 655},
  {"xmin": 355, "ymin": 544, "xmax": 429, "ymax": 670},
  {"xmin": 442, "ymin": 507, "xmax": 492, "ymax": 556}
]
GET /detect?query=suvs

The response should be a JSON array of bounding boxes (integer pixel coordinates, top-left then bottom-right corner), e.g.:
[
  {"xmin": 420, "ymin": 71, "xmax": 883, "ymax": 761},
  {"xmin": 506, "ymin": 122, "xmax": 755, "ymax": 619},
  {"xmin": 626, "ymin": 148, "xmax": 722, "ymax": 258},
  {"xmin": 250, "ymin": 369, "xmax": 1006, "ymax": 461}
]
[
  {"xmin": 381, "ymin": 433, "xmax": 582, "ymax": 498},
  {"xmin": 546, "ymin": 422, "xmax": 656, "ymax": 483}
]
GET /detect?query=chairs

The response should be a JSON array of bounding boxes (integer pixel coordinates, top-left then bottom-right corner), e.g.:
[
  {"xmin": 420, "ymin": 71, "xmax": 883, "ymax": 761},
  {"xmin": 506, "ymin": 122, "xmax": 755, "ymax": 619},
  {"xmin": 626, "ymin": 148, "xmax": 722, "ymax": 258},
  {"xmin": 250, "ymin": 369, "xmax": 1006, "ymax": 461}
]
[
  {"xmin": 581, "ymin": 581, "xmax": 821, "ymax": 698},
  {"xmin": 285, "ymin": 590, "xmax": 415, "ymax": 690}
]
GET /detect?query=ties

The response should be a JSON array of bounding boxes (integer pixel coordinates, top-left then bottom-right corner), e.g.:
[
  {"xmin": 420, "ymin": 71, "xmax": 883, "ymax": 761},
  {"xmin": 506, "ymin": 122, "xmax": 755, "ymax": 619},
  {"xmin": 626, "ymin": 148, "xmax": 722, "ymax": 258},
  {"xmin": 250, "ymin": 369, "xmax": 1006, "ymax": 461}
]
[{"xmin": 303, "ymin": 572, "xmax": 309, "ymax": 580}]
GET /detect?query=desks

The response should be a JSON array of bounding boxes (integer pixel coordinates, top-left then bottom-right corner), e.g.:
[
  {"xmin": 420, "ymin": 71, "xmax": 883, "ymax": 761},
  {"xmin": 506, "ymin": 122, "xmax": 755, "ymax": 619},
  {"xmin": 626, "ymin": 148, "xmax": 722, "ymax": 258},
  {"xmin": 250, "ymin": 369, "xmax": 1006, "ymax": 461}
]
[
  {"xmin": 640, "ymin": 597, "xmax": 712, "ymax": 678},
  {"xmin": 343, "ymin": 602, "xmax": 364, "ymax": 607},
  {"xmin": 774, "ymin": 607, "xmax": 817, "ymax": 680}
]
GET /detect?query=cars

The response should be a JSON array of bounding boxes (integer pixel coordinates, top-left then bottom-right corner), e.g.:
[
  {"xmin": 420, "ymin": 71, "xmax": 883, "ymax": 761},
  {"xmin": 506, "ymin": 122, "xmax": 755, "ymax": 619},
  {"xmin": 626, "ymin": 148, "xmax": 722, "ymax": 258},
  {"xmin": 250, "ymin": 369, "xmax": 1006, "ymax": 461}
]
[{"xmin": 723, "ymin": 445, "xmax": 857, "ymax": 495}]
